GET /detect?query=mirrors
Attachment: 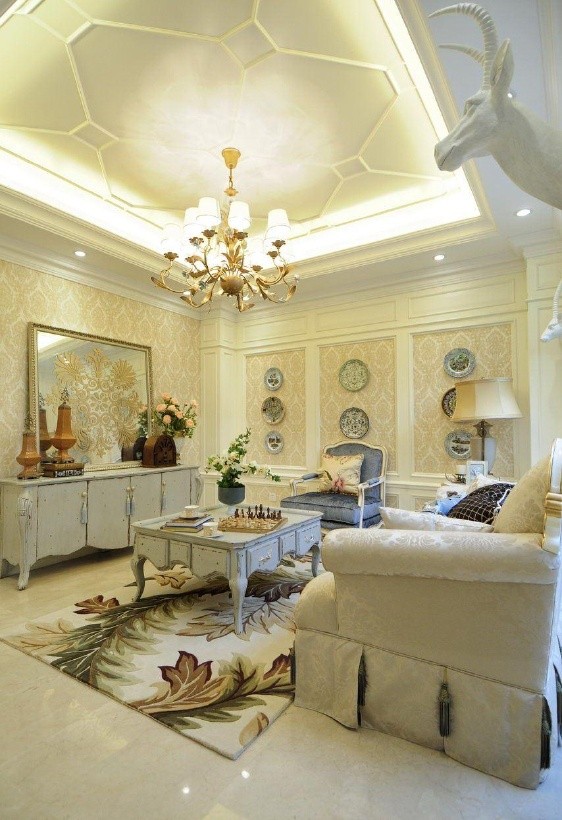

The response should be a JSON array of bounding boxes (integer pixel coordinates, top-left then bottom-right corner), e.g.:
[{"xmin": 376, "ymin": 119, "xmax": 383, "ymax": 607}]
[{"xmin": 24, "ymin": 321, "xmax": 157, "ymax": 473}]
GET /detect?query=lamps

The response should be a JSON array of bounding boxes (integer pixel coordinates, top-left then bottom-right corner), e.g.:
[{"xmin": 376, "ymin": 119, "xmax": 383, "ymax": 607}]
[
  {"xmin": 450, "ymin": 377, "xmax": 524, "ymax": 481},
  {"xmin": 150, "ymin": 145, "xmax": 301, "ymax": 313}
]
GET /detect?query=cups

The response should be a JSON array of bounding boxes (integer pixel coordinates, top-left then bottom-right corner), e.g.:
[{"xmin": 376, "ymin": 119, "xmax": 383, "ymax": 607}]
[
  {"xmin": 203, "ymin": 521, "xmax": 219, "ymax": 537},
  {"xmin": 186, "ymin": 506, "xmax": 199, "ymax": 517}
]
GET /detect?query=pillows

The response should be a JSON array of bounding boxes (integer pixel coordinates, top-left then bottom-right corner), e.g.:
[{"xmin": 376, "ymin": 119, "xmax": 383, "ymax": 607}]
[
  {"xmin": 377, "ymin": 504, "xmax": 494, "ymax": 535},
  {"xmin": 319, "ymin": 450, "xmax": 364, "ymax": 497},
  {"xmin": 445, "ymin": 482, "xmax": 515, "ymax": 524}
]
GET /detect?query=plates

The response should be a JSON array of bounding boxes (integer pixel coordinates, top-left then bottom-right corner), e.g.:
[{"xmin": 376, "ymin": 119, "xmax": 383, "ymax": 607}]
[
  {"xmin": 203, "ymin": 531, "xmax": 222, "ymax": 538},
  {"xmin": 181, "ymin": 514, "xmax": 203, "ymax": 518}
]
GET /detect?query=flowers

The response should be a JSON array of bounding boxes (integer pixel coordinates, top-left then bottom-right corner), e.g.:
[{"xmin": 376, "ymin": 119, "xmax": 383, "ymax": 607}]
[
  {"xmin": 152, "ymin": 390, "xmax": 200, "ymax": 439},
  {"xmin": 202, "ymin": 425, "xmax": 282, "ymax": 488},
  {"xmin": 134, "ymin": 399, "xmax": 150, "ymax": 438}
]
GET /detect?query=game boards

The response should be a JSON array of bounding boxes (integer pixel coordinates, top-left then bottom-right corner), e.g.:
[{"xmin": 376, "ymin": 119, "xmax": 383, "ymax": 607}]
[{"xmin": 217, "ymin": 514, "xmax": 288, "ymax": 534}]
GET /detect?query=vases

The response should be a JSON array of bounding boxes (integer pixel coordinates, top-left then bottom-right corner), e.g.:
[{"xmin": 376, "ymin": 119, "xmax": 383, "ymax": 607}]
[
  {"xmin": 161, "ymin": 431, "xmax": 182, "ymax": 465},
  {"xmin": 217, "ymin": 483, "xmax": 245, "ymax": 515}
]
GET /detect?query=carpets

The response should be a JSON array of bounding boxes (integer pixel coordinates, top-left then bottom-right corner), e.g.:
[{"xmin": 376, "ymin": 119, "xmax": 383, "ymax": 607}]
[{"xmin": 0, "ymin": 547, "xmax": 330, "ymax": 760}]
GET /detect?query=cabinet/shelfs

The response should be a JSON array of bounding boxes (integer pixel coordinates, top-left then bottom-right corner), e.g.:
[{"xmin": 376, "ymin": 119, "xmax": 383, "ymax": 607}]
[{"xmin": 0, "ymin": 462, "xmax": 206, "ymax": 592}]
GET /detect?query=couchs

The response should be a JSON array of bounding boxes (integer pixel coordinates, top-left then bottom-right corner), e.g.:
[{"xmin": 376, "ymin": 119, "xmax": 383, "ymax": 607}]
[{"xmin": 288, "ymin": 438, "xmax": 562, "ymax": 793}]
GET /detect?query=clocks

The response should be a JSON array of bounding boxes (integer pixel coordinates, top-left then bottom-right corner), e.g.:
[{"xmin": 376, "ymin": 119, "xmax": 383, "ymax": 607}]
[{"xmin": 142, "ymin": 434, "xmax": 178, "ymax": 469}]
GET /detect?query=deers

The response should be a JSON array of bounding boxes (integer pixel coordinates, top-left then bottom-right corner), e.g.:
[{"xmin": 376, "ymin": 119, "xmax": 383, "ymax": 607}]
[
  {"xmin": 539, "ymin": 281, "xmax": 562, "ymax": 342},
  {"xmin": 427, "ymin": 2, "xmax": 562, "ymax": 211}
]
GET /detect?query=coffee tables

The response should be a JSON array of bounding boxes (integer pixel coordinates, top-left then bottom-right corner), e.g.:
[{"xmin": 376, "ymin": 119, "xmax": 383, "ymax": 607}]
[{"xmin": 128, "ymin": 503, "xmax": 325, "ymax": 634}]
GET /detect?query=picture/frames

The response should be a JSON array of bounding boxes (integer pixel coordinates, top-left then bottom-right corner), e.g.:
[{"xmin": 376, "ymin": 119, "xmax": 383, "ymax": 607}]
[{"xmin": 466, "ymin": 459, "xmax": 488, "ymax": 486}]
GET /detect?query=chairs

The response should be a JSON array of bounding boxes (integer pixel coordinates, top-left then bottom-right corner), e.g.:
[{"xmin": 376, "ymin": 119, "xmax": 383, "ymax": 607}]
[{"xmin": 279, "ymin": 440, "xmax": 388, "ymax": 538}]
